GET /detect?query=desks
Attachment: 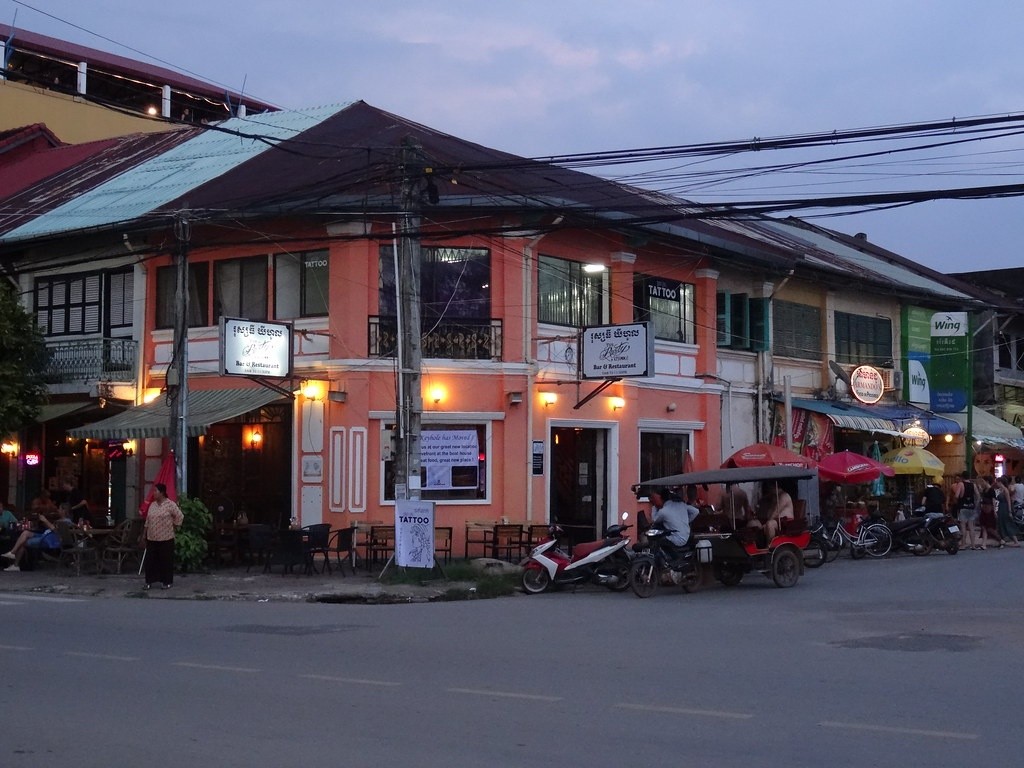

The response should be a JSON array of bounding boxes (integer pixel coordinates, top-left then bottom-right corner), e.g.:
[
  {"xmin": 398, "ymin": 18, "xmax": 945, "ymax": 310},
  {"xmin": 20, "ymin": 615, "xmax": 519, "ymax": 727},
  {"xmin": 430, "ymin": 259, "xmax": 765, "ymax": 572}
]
[
  {"xmin": 213, "ymin": 524, "xmax": 270, "ymax": 565},
  {"xmin": 70, "ymin": 527, "xmax": 122, "ymax": 573},
  {"xmin": 474, "ymin": 522, "xmax": 495, "ymax": 528}
]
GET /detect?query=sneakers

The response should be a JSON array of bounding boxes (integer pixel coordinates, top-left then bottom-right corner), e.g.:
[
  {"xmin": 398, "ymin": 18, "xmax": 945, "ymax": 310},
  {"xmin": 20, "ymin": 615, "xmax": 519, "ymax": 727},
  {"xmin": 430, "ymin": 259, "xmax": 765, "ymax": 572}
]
[
  {"xmin": 1, "ymin": 551, "xmax": 15, "ymax": 559},
  {"xmin": 3, "ymin": 564, "xmax": 20, "ymax": 571}
]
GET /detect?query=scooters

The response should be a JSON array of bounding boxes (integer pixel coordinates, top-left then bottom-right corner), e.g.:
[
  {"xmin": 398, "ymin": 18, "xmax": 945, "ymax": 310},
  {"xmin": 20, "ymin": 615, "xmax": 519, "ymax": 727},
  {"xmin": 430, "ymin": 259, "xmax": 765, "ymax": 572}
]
[
  {"xmin": 850, "ymin": 505, "xmax": 963, "ymax": 559},
  {"xmin": 600, "ymin": 509, "xmax": 705, "ymax": 598},
  {"xmin": 520, "ymin": 515, "xmax": 633, "ymax": 595}
]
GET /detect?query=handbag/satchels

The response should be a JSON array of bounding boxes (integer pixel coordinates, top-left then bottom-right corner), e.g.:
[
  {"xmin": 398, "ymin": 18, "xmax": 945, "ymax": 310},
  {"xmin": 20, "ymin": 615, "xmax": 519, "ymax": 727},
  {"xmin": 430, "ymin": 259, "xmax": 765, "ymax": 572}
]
[{"xmin": 981, "ymin": 504, "xmax": 993, "ymax": 514}]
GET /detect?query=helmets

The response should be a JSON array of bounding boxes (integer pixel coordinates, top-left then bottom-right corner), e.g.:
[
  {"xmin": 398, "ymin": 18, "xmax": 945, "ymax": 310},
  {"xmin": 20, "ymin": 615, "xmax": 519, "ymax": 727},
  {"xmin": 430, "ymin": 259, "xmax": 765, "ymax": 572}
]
[{"xmin": 932, "ymin": 476, "xmax": 944, "ymax": 488}]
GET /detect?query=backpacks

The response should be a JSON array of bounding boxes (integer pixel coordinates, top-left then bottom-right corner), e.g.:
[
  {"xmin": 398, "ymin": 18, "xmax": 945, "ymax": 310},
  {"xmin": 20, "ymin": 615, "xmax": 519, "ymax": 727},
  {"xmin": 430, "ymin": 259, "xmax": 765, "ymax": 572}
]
[{"xmin": 962, "ymin": 480, "xmax": 975, "ymax": 505}]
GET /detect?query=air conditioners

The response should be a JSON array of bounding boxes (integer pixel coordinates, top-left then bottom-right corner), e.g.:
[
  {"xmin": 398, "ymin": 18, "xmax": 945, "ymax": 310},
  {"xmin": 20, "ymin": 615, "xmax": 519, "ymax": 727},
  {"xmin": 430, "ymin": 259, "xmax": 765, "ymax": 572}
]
[{"xmin": 882, "ymin": 368, "xmax": 903, "ymax": 391}]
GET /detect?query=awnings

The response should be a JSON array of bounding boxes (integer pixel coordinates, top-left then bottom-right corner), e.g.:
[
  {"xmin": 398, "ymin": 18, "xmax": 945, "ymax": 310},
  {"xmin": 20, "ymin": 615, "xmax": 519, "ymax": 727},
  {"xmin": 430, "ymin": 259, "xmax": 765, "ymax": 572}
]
[
  {"xmin": 847, "ymin": 400, "xmax": 963, "ymax": 434},
  {"xmin": 11, "ymin": 398, "xmax": 95, "ymax": 423},
  {"xmin": 913, "ymin": 402, "xmax": 1024, "ymax": 449},
  {"xmin": 772, "ymin": 394, "xmax": 895, "ymax": 431},
  {"xmin": 66, "ymin": 387, "xmax": 297, "ymax": 438}
]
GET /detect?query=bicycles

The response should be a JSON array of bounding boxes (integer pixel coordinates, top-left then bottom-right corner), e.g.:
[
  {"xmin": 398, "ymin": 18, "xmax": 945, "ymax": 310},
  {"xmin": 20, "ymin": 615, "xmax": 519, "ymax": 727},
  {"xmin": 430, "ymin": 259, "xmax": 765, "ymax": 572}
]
[{"xmin": 818, "ymin": 513, "xmax": 892, "ymax": 562}]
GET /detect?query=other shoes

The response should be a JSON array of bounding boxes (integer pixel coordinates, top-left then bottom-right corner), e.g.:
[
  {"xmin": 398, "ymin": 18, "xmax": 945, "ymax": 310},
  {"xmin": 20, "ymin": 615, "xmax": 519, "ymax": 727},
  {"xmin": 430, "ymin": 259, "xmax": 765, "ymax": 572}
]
[
  {"xmin": 143, "ymin": 583, "xmax": 151, "ymax": 590},
  {"xmin": 1000, "ymin": 543, "xmax": 1005, "ymax": 549},
  {"xmin": 971, "ymin": 546, "xmax": 975, "ymax": 549},
  {"xmin": 980, "ymin": 545, "xmax": 987, "ymax": 550},
  {"xmin": 1012, "ymin": 544, "xmax": 1020, "ymax": 548},
  {"xmin": 161, "ymin": 584, "xmax": 173, "ymax": 588},
  {"xmin": 959, "ymin": 546, "xmax": 965, "ymax": 550}
]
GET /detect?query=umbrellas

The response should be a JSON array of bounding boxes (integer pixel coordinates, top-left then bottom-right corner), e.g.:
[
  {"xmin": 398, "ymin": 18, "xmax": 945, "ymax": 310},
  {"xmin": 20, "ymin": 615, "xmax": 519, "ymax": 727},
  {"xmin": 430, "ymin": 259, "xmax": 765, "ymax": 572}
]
[
  {"xmin": 870, "ymin": 441, "xmax": 885, "ymax": 509},
  {"xmin": 883, "ymin": 447, "xmax": 946, "ymax": 514},
  {"xmin": 721, "ymin": 442, "xmax": 816, "ymax": 468},
  {"xmin": 139, "ymin": 449, "xmax": 176, "ymax": 574},
  {"xmin": 819, "ymin": 449, "xmax": 895, "ymax": 516}
]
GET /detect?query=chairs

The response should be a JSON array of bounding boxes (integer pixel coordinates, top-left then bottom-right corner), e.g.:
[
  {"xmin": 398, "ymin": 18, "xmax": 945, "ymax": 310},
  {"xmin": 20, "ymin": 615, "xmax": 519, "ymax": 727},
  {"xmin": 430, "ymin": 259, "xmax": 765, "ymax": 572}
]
[
  {"xmin": 776, "ymin": 499, "xmax": 807, "ymax": 533},
  {"xmin": 0, "ymin": 517, "xmax": 145, "ymax": 572},
  {"xmin": 203, "ymin": 520, "xmax": 557, "ymax": 573}
]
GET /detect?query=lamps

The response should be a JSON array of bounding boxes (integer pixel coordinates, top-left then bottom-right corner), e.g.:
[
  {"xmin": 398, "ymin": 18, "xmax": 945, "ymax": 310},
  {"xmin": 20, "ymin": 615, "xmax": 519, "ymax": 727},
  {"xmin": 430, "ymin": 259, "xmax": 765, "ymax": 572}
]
[
  {"xmin": 614, "ymin": 398, "xmax": 625, "ymax": 410},
  {"xmin": 544, "ymin": 393, "xmax": 558, "ymax": 406},
  {"xmin": 667, "ymin": 403, "xmax": 676, "ymax": 412},
  {"xmin": 431, "ymin": 387, "xmax": 444, "ymax": 402},
  {"xmin": 251, "ymin": 429, "xmax": 261, "ymax": 446},
  {"xmin": 304, "ymin": 384, "xmax": 319, "ymax": 400}
]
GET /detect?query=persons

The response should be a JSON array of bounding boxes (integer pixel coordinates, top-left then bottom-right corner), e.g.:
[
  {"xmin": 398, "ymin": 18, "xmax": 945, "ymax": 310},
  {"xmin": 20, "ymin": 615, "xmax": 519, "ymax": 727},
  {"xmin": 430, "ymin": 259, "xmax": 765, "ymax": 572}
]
[
  {"xmin": 824, "ymin": 483, "xmax": 842, "ymax": 515},
  {"xmin": 141, "ymin": 483, "xmax": 184, "ymax": 589},
  {"xmin": 922, "ymin": 484, "xmax": 944, "ymax": 520},
  {"xmin": 946, "ymin": 471, "xmax": 1024, "ymax": 550},
  {"xmin": 854, "ymin": 486, "xmax": 886, "ymax": 516},
  {"xmin": 687, "ymin": 484, "xmax": 709, "ymax": 532},
  {"xmin": 651, "ymin": 488, "xmax": 699, "ymax": 585},
  {"xmin": 894, "ymin": 502, "xmax": 906, "ymax": 522},
  {"xmin": 716, "ymin": 484, "xmax": 751, "ymax": 531},
  {"xmin": 747, "ymin": 480, "xmax": 794, "ymax": 572},
  {"xmin": 0, "ymin": 480, "xmax": 91, "ymax": 571},
  {"xmin": 974, "ymin": 447, "xmax": 995, "ymax": 478}
]
